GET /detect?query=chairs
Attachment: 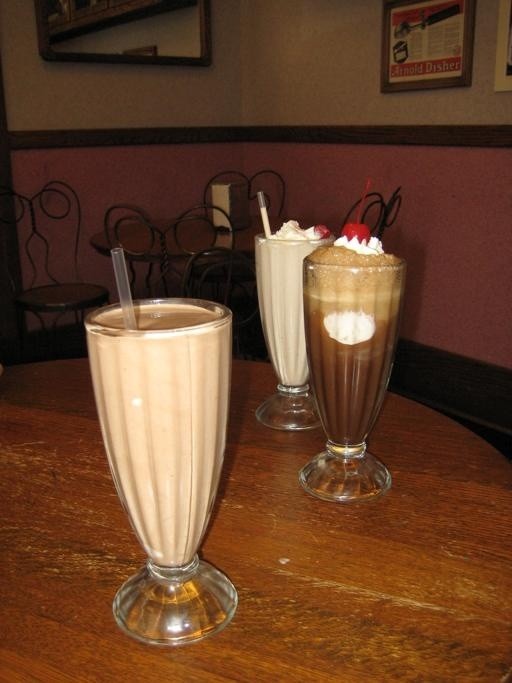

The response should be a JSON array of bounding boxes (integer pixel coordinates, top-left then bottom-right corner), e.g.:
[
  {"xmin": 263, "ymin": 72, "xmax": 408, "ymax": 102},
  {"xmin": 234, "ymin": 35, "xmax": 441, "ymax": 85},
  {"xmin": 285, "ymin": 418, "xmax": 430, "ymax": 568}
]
[
  {"xmin": 0, "ymin": 178, "xmax": 112, "ymax": 359},
  {"xmin": 178, "ymin": 244, "xmax": 261, "ymax": 332},
  {"xmin": 109, "ymin": 204, "xmax": 237, "ymax": 302},
  {"xmin": 333, "ymin": 184, "xmax": 405, "ymax": 242},
  {"xmin": 199, "ymin": 167, "xmax": 288, "ymax": 227}
]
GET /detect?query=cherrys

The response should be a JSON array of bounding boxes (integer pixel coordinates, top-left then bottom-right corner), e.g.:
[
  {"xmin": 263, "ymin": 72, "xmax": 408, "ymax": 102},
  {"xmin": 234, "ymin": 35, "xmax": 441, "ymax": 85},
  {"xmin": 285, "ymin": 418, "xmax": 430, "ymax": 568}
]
[
  {"xmin": 341, "ymin": 179, "xmax": 372, "ymax": 244},
  {"xmin": 315, "ymin": 223, "xmax": 331, "ymax": 239}
]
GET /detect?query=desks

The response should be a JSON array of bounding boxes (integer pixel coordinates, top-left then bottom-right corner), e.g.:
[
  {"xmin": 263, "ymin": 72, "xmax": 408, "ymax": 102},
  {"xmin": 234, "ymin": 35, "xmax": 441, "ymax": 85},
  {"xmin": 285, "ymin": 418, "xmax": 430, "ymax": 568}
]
[{"xmin": 87, "ymin": 209, "xmax": 295, "ymax": 306}]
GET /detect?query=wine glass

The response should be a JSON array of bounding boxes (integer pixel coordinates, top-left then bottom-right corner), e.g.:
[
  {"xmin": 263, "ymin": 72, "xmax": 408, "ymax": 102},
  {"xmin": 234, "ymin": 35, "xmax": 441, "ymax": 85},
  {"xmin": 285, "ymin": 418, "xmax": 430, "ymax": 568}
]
[{"xmin": 299, "ymin": 258, "xmax": 406, "ymax": 504}]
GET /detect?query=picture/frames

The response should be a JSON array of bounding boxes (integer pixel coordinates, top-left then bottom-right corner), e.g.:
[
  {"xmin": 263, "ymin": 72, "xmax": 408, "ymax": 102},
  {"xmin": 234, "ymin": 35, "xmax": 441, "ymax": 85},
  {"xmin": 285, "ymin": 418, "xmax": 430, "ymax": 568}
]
[
  {"xmin": 379, "ymin": 0, "xmax": 477, "ymax": 94},
  {"xmin": 491, "ymin": 1, "xmax": 512, "ymax": 94},
  {"xmin": 32, "ymin": 0, "xmax": 213, "ymax": 68}
]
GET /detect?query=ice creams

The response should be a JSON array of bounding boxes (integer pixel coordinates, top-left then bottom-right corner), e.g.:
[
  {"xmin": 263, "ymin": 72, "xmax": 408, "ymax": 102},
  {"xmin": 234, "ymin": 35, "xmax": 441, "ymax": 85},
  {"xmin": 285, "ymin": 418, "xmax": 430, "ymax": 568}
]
[{"xmin": 301, "ymin": 179, "xmax": 406, "ymax": 502}]
